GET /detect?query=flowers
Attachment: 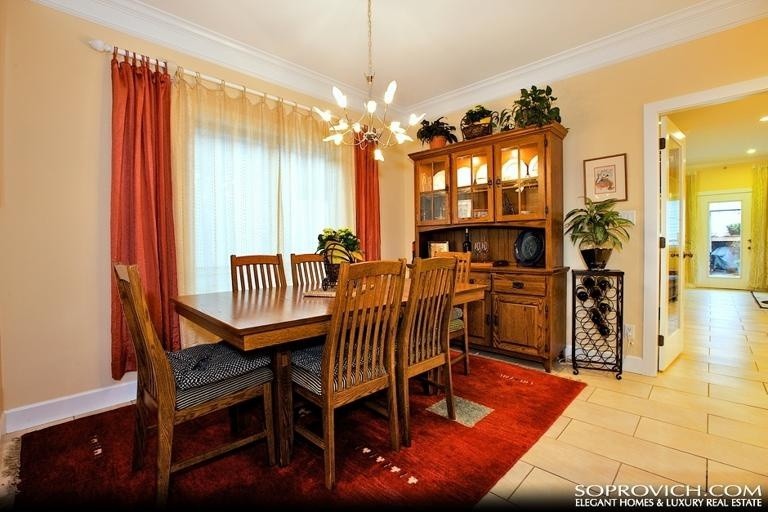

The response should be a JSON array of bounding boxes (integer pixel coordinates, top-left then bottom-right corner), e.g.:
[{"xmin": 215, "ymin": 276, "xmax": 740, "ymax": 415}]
[{"xmin": 314, "ymin": 226, "xmax": 365, "ymax": 265}]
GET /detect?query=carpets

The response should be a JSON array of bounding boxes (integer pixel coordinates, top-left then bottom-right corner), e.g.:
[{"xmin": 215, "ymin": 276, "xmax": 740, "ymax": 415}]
[
  {"xmin": 752, "ymin": 292, "xmax": 767, "ymax": 308},
  {"xmin": 750, "ymin": 290, "xmax": 768, "ymax": 309},
  {"xmin": 17, "ymin": 345, "xmax": 588, "ymax": 507}
]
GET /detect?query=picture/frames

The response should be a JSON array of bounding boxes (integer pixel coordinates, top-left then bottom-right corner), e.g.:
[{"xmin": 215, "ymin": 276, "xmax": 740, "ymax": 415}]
[
  {"xmin": 427, "ymin": 240, "xmax": 449, "ymax": 259},
  {"xmin": 582, "ymin": 152, "xmax": 629, "ymax": 207}
]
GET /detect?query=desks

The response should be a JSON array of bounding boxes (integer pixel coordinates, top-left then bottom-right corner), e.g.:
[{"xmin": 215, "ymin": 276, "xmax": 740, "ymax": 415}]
[{"xmin": 173, "ymin": 283, "xmax": 492, "ymax": 467}]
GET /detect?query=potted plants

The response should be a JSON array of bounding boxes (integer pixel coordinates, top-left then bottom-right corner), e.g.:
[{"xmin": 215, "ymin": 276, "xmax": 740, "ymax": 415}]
[
  {"xmin": 459, "ymin": 106, "xmax": 499, "ymax": 140},
  {"xmin": 502, "ymin": 83, "xmax": 562, "ymax": 132},
  {"xmin": 562, "ymin": 194, "xmax": 637, "ymax": 269}
]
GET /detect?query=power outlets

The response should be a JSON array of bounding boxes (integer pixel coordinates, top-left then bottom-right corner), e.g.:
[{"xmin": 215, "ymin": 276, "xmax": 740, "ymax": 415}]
[{"xmin": 624, "ymin": 324, "xmax": 635, "ymax": 343}]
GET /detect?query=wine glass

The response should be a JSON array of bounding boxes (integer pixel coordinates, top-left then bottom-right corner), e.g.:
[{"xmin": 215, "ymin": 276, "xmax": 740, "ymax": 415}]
[
  {"xmin": 480, "ymin": 240, "xmax": 490, "ymax": 263},
  {"xmin": 473, "ymin": 242, "xmax": 480, "ymax": 263}
]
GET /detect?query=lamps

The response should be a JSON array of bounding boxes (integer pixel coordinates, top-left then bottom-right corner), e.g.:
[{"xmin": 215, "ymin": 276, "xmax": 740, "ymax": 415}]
[{"xmin": 311, "ymin": 0, "xmax": 427, "ymax": 162}]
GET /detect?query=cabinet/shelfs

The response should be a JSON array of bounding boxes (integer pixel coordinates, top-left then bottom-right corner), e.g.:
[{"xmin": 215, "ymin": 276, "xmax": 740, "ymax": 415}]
[
  {"xmin": 570, "ymin": 269, "xmax": 625, "ymax": 384},
  {"xmin": 408, "ymin": 117, "xmax": 568, "ymax": 373}
]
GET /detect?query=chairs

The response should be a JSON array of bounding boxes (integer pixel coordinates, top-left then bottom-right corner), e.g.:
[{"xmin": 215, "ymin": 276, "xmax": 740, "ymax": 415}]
[
  {"xmin": 113, "ymin": 262, "xmax": 279, "ymax": 507},
  {"xmin": 290, "ymin": 253, "xmax": 325, "ymax": 288},
  {"xmin": 229, "ymin": 253, "xmax": 288, "ymax": 290},
  {"xmin": 289, "ymin": 257, "xmax": 408, "ymax": 491},
  {"xmin": 433, "ymin": 250, "xmax": 471, "ymax": 395},
  {"xmin": 392, "ymin": 253, "xmax": 459, "ymax": 448}
]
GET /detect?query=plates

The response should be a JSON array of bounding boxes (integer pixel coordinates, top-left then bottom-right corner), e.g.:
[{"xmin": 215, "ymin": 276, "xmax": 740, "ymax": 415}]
[
  {"xmin": 514, "ymin": 228, "xmax": 544, "ymax": 267},
  {"xmin": 528, "ymin": 154, "xmax": 538, "ymax": 177},
  {"xmin": 476, "ymin": 163, "xmax": 487, "ymax": 185},
  {"xmin": 433, "ymin": 169, "xmax": 446, "ymax": 191},
  {"xmin": 501, "ymin": 158, "xmax": 527, "ymax": 181},
  {"xmin": 457, "ymin": 165, "xmax": 474, "ymax": 188}
]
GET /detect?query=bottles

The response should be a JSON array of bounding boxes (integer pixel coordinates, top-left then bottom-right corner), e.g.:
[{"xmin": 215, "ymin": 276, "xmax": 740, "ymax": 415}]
[{"xmin": 463, "ymin": 228, "xmax": 472, "ymax": 252}]
